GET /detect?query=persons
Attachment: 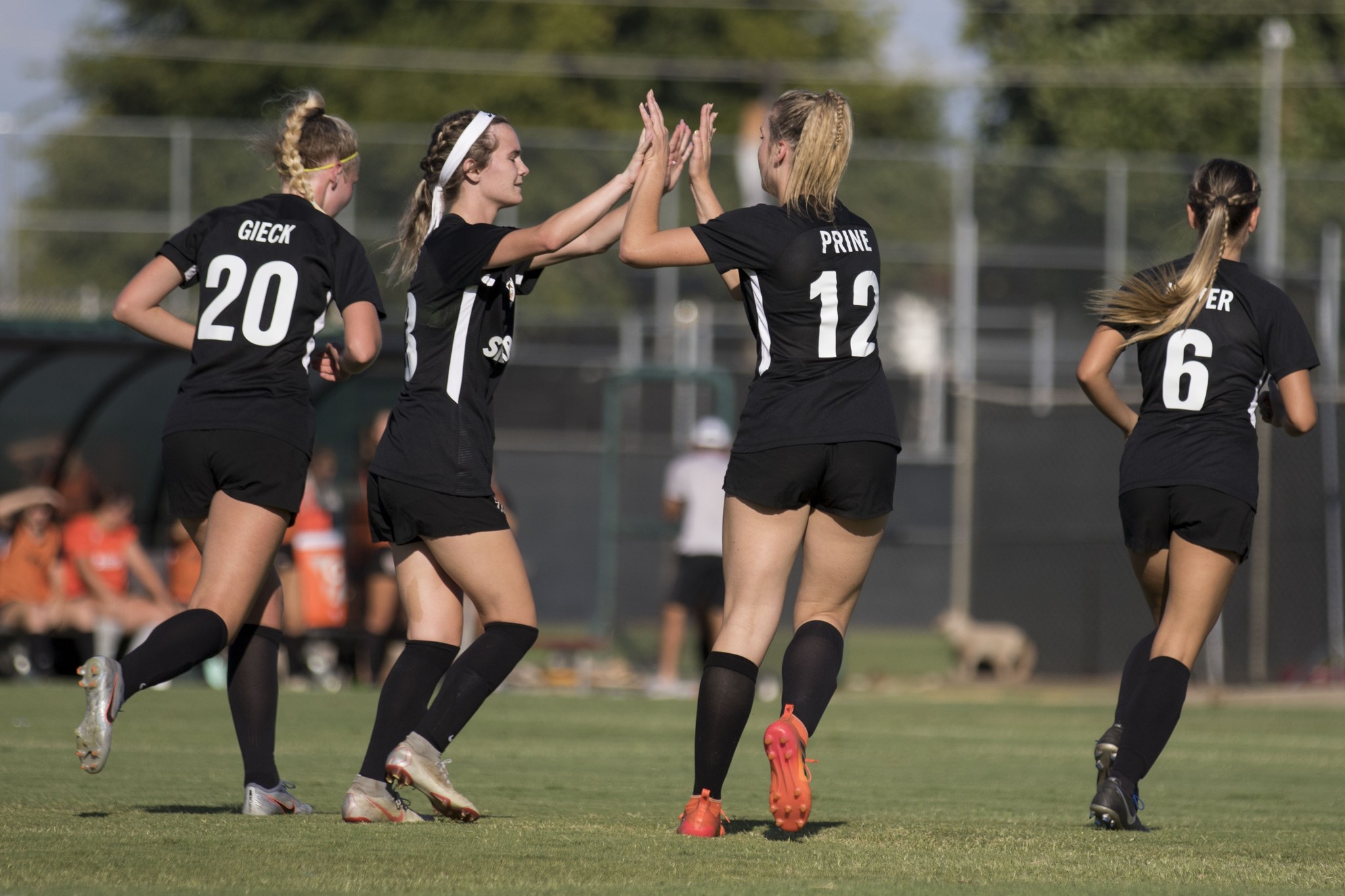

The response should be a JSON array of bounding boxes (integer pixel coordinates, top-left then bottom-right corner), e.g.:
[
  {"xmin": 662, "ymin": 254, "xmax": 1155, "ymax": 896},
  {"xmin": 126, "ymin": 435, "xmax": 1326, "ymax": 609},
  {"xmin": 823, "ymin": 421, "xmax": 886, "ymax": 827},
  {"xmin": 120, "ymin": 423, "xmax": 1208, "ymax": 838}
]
[
  {"xmin": 644, "ymin": 416, "xmax": 733, "ymax": 694},
  {"xmin": 0, "ymin": 404, "xmax": 407, "ymax": 694},
  {"xmin": 338, "ymin": 103, "xmax": 697, "ymax": 824},
  {"xmin": 76, "ymin": 86, "xmax": 382, "ymax": 817},
  {"xmin": 1080, "ymin": 157, "xmax": 1315, "ymax": 833},
  {"xmin": 619, "ymin": 86, "xmax": 903, "ymax": 839}
]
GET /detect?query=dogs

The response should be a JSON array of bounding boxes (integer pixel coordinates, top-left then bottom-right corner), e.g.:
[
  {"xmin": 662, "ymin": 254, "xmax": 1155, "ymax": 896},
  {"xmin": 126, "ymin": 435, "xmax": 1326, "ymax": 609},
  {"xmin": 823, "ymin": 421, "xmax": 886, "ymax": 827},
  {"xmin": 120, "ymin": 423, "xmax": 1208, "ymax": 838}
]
[{"xmin": 940, "ymin": 610, "xmax": 1038, "ymax": 685}]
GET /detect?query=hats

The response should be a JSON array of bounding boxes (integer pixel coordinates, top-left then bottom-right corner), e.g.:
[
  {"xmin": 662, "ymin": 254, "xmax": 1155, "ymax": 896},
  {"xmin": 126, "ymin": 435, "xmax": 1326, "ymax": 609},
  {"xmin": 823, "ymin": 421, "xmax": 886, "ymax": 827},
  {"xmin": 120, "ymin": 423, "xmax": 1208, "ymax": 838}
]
[{"xmin": 688, "ymin": 415, "xmax": 734, "ymax": 450}]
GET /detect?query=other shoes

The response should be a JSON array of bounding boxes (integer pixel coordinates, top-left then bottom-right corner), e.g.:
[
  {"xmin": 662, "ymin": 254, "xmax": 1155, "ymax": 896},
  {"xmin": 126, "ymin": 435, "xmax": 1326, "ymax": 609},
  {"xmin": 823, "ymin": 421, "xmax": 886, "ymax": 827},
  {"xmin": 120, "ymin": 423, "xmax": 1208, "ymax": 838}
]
[{"xmin": 649, "ymin": 676, "xmax": 699, "ymax": 699}]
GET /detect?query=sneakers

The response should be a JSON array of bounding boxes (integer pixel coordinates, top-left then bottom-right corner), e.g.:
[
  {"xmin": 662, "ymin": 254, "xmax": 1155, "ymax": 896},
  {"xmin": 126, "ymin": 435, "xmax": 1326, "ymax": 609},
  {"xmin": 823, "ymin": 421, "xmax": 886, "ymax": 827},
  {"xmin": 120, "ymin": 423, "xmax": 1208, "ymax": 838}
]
[
  {"xmin": 342, "ymin": 774, "xmax": 435, "ymax": 823},
  {"xmin": 1088, "ymin": 772, "xmax": 1149, "ymax": 832},
  {"xmin": 763, "ymin": 703, "xmax": 819, "ymax": 834},
  {"xmin": 385, "ymin": 731, "xmax": 481, "ymax": 823},
  {"xmin": 242, "ymin": 775, "xmax": 313, "ymax": 818},
  {"xmin": 73, "ymin": 654, "xmax": 126, "ymax": 774},
  {"xmin": 677, "ymin": 788, "xmax": 730, "ymax": 838},
  {"xmin": 1093, "ymin": 725, "xmax": 1126, "ymax": 794}
]
